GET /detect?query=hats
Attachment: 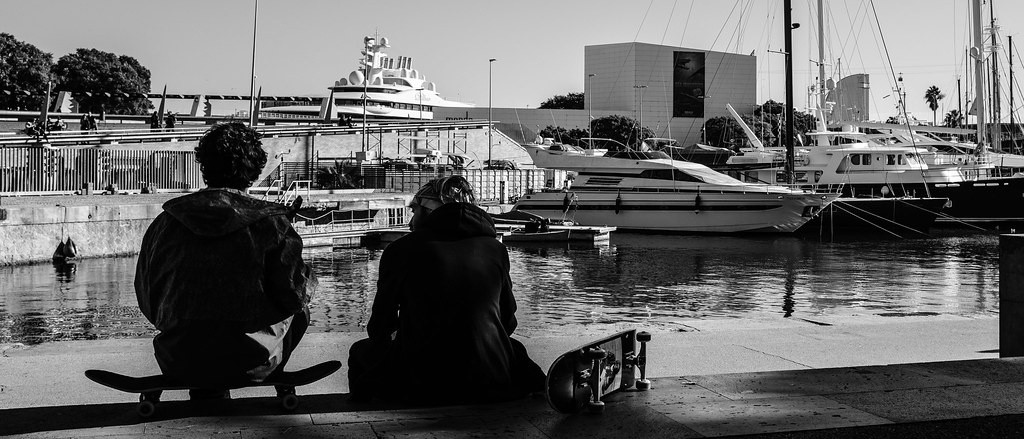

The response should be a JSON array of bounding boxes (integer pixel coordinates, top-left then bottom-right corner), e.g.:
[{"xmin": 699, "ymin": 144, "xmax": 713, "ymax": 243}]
[{"xmin": 409, "ymin": 176, "xmax": 475, "ymax": 216}]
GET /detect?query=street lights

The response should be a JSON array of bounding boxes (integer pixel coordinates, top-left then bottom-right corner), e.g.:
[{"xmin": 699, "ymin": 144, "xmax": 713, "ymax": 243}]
[
  {"xmin": 633, "ymin": 84, "xmax": 649, "ymax": 139},
  {"xmin": 252, "ymin": 75, "xmax": 258, "ymax": 108},
  {"xmin": 756, "ymin": 104, "xmax": 764, "ymax": 144},
  {"xmin": 930, "ymin": 92, "xmax": 936, "ymax": 126},
  {"xmin": 415, "ymin": 88, "xmax": 424, "ymax": 120},
  {"xmin": 361, "ymin": 37, "xmax": 376, "ymax": 152},
  {"xmin": 588, "ymin": 73, "xmax": 597, "ymax": 149},
  {"xmin": 487, "ymin": 56, "xmax": 499, "ymax": 169},
  {"xmin": 698, "ymin": 95, "xmax": 712, "ymax": 145}
]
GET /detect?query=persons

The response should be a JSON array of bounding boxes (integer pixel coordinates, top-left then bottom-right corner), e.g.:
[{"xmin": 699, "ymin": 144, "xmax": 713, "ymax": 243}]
[
  {"xmin": 57, "ymin": 106, "xmax": 61, "ymax": 113},
  {"xmin": 165, "ymin": 111, "xmax": 176, "ymax": 132},
  {"xmin": 150, "ymin": 111, "xmax": 158, "ymax": 132},
  {"xmin": 25, "ymin": 117, "xmax": 63, "ymax": 140},
  {"xmin": 338, "ymin": 114, "xmax": 354, "ymax": 134},
  {"xmin": 80, "ymin": 111, "xmax": 98, "ymax": 144},
  {"xmin": 347, "ymin": 176, "xmax": 549, "ymax": 413},
  {"xmin": 133, "ymin": 122, "xmax": 310, "ymax": 404}
]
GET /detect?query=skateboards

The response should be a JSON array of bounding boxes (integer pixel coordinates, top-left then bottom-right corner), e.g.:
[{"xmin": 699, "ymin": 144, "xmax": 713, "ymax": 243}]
[
  {"xmin": 542, "ymin": 327, "xmax": 652, "ymax": 418},
  {"xmin": 82, "ymin": 358, "xmax": 342, "ymax": 419}
]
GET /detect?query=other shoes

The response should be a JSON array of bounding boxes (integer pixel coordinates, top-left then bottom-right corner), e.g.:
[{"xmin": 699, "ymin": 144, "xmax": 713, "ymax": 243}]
[{"xmin": 188, "ymin": 389, "xmax": 232, "ymax": 399}]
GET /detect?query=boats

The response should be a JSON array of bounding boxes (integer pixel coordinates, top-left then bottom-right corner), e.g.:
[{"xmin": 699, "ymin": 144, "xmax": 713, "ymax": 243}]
[{"xmin": 508, "ymin": 107, "xmax": 846, "ymax": 238}]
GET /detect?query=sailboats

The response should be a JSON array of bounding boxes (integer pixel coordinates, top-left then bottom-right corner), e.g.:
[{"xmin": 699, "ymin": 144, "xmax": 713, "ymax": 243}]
[{"xmin": 585, "ymin": 0, "xmax": 1024, "ymax": 238}]
